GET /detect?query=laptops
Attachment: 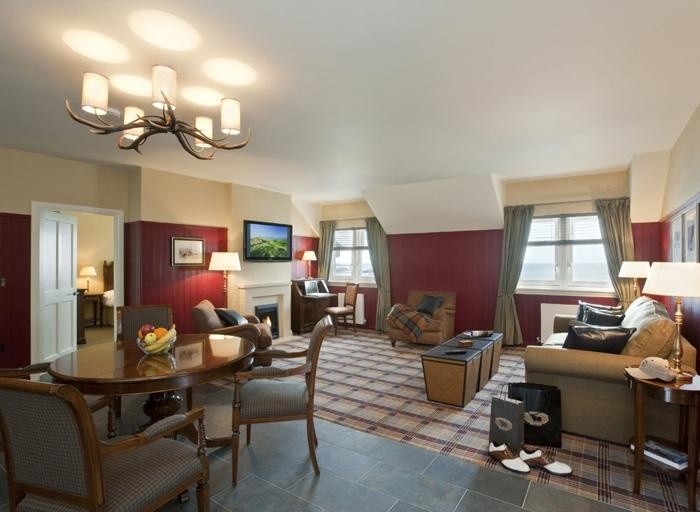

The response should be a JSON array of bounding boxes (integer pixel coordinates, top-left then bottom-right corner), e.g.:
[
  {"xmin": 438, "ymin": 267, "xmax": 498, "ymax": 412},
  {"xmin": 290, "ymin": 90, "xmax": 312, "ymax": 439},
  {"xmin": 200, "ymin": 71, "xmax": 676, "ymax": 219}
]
[{"xmin": 303, "ymin": 280, "xmax": 329, "ymax": 296}]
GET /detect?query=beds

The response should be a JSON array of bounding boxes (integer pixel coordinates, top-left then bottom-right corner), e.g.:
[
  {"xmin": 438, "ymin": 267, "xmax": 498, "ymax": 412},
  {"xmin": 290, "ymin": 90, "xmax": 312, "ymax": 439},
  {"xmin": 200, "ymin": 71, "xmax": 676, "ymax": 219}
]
[{"xmin": 103, "ymin": 260, "xmax": 114, "ymax": 328}]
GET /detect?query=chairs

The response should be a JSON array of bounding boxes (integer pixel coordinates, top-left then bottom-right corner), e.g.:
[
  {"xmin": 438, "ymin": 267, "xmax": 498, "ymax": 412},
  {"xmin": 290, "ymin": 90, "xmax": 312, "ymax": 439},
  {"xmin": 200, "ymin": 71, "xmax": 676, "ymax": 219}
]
[
  {"xmin": 232, "ymin": 314, "xmax": 333, "ymax": 487},
  {"xmin": 0, "ymin": 378, "xmax": 210, "ymax": 512},
  {"xmin": 0, "ymin": 362, "xmax": 121, "ymax": 439},
  {"xmin": 325, "ymin": 282, "xmax": 359, "ymax": 336},
  {"xmin": 113, "ymin": 304, "xmax": 192, "ymax": 418}
]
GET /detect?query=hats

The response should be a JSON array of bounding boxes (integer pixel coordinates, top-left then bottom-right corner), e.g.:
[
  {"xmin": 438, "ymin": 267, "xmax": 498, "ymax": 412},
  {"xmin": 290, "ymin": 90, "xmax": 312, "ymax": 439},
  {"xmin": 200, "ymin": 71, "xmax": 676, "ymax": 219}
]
[{"xmin": 624, "ymin": 355, "xmax": 677, "ymax": 383}]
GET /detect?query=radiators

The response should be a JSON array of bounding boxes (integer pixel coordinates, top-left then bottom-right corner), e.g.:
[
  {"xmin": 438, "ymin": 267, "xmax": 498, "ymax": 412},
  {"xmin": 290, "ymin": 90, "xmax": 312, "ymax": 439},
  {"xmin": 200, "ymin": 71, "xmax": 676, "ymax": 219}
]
[
  {"xmin": 536, "ymin": 303, "xmax": 579, "ymax": 344},
  {"xmin": 337, "ymin": 292, "xmax": 367, "ymax": 325}
]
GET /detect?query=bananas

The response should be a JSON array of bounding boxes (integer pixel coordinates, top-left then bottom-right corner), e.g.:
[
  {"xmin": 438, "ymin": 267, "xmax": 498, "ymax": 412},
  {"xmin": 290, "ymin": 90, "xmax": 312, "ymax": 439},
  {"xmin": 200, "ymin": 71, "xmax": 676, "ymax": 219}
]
[{"xmin": 144, "ymin": 324, "xmax": 176, "ymax": 352}]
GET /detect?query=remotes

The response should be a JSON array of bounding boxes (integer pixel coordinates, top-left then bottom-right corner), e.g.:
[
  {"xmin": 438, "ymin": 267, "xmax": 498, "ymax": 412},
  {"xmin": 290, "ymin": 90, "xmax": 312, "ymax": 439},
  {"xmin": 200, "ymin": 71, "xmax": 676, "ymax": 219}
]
[{"xmin": 444, "ymin": 350, "xmax": 468, "ymax": 354}]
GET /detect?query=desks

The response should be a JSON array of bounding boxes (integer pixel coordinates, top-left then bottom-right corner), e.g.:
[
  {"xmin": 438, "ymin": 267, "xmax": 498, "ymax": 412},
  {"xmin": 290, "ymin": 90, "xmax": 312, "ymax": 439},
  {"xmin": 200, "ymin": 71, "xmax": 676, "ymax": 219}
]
[
  {"xmin": 625, "ymin": 364, "xmax": 700, "ymax": 512},
  {"xmin": 48, "ymin": 334, "xmax": 255, "ymax": 487},
  {"xmin": 84, "ymin": 292, "xmax": 104, "ymax": 327}
]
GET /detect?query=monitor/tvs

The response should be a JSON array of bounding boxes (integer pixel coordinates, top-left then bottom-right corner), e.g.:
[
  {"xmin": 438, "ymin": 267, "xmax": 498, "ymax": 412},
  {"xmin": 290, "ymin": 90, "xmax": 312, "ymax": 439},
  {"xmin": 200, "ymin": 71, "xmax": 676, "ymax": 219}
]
[{"xmin": 243, "ymin": 219, "xmax": 292, "ymax": 262}]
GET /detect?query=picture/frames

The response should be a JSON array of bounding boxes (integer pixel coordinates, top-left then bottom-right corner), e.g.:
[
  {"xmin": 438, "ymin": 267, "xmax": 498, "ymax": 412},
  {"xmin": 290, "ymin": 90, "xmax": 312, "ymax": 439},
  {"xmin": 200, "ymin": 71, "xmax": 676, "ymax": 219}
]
[
  {"xmin": 171, "ymin": 237, "xmax": 205, "ymax": 267},
  {"xmin": 304, "ymin": 280, "xmax": 319, "ymax": 295}
]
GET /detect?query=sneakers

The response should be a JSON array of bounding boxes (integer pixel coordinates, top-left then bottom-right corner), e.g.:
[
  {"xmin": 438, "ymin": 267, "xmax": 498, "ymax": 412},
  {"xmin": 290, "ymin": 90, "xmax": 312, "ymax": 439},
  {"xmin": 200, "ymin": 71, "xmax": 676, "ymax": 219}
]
[
  {"xmin": 519, "ymin": 447, "xmax": 575, "ymax": 476},
  {"xmin": 487, "ymin": 438, "xmax": 529, "ymax": 474}
]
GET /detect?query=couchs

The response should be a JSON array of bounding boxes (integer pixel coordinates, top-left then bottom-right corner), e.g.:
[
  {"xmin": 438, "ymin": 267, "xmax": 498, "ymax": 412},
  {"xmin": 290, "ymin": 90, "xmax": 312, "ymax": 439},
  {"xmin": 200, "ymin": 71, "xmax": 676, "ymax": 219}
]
[
  {"xmin": 193, "ymin": 300, "xmax": 272, "ymax": 369},
  {"xmin": 525, "ymin": 296, "xmax": 696, "ymax": 446},
  {"xmin": 387, "ymin": 290, "xmax": 456, "ymax": 347}
]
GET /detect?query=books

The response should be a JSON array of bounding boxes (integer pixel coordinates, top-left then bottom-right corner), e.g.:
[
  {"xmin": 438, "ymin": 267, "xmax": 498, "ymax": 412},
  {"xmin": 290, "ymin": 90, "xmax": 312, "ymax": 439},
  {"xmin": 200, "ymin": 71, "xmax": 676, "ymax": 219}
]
[{"xmin": 631, "ymin": 438, "xmax": 687, "ymax": 470}]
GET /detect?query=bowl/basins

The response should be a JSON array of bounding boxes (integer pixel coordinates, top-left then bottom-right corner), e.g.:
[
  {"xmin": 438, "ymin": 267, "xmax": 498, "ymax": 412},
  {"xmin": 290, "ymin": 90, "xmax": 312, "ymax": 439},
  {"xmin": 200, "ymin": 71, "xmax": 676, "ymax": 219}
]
[{"xmin": 136, "ymin": 336, "xmax": 175, "ymax": 356}]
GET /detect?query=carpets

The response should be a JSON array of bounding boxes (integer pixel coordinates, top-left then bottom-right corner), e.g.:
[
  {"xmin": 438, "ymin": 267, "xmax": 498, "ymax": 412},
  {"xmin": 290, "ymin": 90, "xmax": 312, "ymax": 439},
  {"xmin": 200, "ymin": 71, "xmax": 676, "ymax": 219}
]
[{"xmin": 206, "ymin": 324, "xmax": 700, "ymax": 512}]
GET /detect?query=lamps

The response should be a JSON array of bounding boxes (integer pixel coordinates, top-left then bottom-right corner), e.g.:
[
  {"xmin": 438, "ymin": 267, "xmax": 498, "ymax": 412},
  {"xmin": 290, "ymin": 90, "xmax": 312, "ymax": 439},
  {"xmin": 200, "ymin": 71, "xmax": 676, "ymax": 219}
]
[
  {"xmin": 208, "ymin": 252, "xmax": 242, "ymax": 293},
  {"xmin": 64, "ymin": 64, "xmax": 253, "ymax": 160},
  {"xmin": 618, "ymin": 262, "xmax": 651, "ymax": 299},
  {"xmin": 302, "ymin": 251, "xmax": 317, "ymax": 279},
  {"xmin": 642, "ymin": 262, "xmax": 700, "ymax": 381},
  {"xmin": 79, "ymin": 266, "xmax": 97, "ymax": 293}
]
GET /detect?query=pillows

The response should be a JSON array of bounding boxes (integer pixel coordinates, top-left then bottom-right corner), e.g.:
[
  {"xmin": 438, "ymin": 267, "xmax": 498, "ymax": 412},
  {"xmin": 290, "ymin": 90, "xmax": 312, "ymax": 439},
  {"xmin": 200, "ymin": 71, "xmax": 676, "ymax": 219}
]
[
  {"xmin": 416, "ymin": 295, "xmax": 445, "ymax": 315},
  {"xmin": 214, "ymin": 308, "xmax": 248, "ymax": 326},
  {"xmin": 563, "ymin": 299, "xmax": 637, "ymax": 354}
]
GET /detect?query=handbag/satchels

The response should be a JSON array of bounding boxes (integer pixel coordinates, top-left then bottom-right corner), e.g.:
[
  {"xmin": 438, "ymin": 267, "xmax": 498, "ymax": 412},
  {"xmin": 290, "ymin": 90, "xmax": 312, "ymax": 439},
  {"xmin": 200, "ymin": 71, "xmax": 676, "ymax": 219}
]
[
  {"xmin": 506, "ymin": 380, "xmax": 563, "ymax": 449},
  {"xmin": 488, "ymin": 381, "xmax": 528, "ymax": 451}
]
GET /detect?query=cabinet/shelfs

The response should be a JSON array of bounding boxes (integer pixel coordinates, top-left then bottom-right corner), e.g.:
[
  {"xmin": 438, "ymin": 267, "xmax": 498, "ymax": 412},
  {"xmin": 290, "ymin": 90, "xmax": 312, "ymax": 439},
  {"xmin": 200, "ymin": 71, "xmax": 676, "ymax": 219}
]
[{"xmin": 292, "ymin": 278, "xmax": 338, "ymax": 335}]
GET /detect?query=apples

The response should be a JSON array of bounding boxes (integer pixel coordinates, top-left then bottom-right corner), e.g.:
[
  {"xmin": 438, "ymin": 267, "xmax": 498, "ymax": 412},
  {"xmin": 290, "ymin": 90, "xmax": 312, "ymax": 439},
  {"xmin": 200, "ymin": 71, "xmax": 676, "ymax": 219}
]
[{"xmin": 137, "ymin": 325, "xmax": 156, "ymax": 347}]
